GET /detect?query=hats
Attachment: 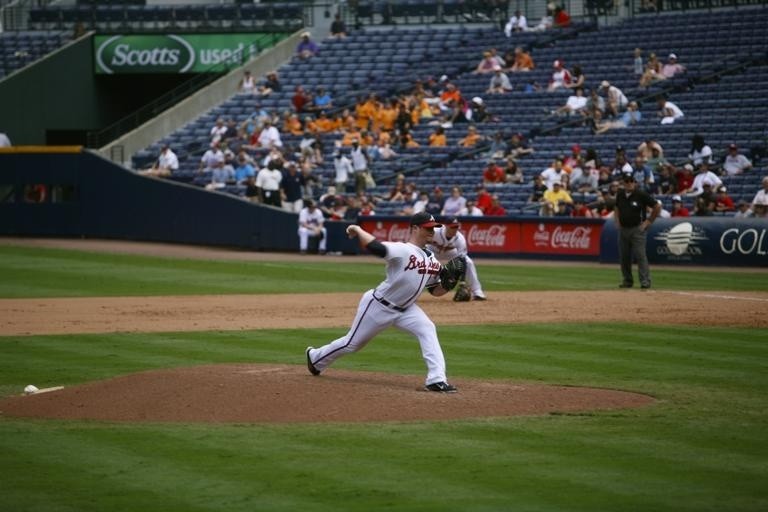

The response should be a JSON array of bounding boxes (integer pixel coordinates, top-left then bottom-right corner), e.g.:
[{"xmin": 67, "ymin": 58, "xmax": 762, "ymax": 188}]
[
  {"xmin": 493, "ymin": 65, "xmax": 501, "ymax": 72},
  {"xmin": 683, "ymin": 164, "xmax": 696, "ymax": 173},
  {"xmin": 411, "ymin": 211, "xmax": 442, "ymax": 229},
  {"xmin": 728, "ymin": 143, "xmax": 738, "ymax": 151},
  {"xmin": 445, "ymin": 216, "xmax": 460, "ymax": 228},
  {"xmin": 622, "ymin": 172, "xmax": 634, "ymax": 181},
  {"xmin": 671, "ymin": 195, "xmax": 682, "ymax": 202},
  {"xmin": 717, "ymin": 185, "xmax": 728, "ymax": 194},
  {"xmin": 552, "ymin": 180, "xmax": 563, "ymax": 186}
]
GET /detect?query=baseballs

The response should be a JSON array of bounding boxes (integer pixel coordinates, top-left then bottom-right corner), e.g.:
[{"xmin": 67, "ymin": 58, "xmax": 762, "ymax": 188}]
[{"xmin": 24, "ymin": 385, "xmax": 35, "ymax": 394}]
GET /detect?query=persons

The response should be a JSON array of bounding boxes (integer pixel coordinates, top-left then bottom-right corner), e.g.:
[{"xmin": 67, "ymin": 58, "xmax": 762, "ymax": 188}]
[
  {"xmin": 421, "ymin": 215, "xmax": 485, "ymax": 303},
  {"xmin": 0, "ymin": 130, "xmax": 13, "ymax": 149},
  {"xmin": 613, "ymin": 170, "xmax": 662, "ymax": 289},
  {"xmin": 149, "ymin": 2, "xmax": 768, "ymax": 217},
  {"xmin": 304, "ymin": 211, "xmax": 460, "ymax": 391},
  {"xmin": 297, "ymin": 199, "xmax": 328, "ymax": 257}
]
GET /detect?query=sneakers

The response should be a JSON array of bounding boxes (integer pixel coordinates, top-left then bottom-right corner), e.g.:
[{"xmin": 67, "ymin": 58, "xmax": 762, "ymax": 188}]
[
  {"xmin": 424, "ymin": 381, "xmax": 457, "ymax": 392},
  {"xmin": 473, "ymin": 296, "xmax": 487, "ymax": 301},
  {"xmin": 305, "ymin": 347, "xmax": 320, "ymax": 378},
  {"xmin": 641, "ymin": 283, "xmax": 650, "ymax": 288},
  {"xmin": 619, "ymin": 281, "xmax": 633, "ymax": 287}
]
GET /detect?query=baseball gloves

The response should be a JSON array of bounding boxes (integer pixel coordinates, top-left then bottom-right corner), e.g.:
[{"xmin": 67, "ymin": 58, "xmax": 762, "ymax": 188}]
[
  {"xmin": 441, "ymin": 256, "xmax": 464, "ymax": 291},
  {"xmin": 454, "ymin": 289, "xmax": 472, "ymax": 302}
]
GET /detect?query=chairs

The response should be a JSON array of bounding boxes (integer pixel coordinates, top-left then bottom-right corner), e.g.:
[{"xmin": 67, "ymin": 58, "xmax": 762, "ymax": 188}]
[{"xmin": 1, "ymin": 2, "xmax": 131, "ymax": 83}]
